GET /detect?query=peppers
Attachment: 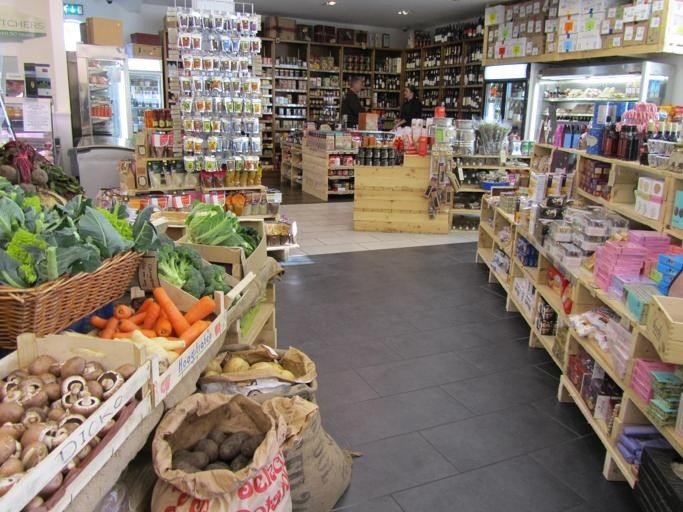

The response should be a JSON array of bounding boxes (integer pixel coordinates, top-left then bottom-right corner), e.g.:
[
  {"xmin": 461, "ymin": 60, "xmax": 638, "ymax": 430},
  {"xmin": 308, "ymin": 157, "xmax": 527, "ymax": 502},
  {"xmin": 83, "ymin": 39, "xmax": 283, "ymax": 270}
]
[
  {"xmin": 173, "ymin": 427, "xmax": 263, "ymax": 479},
  {"xmin": 207, "ymin": 357, "xmax": 295, "ymax": 383}
]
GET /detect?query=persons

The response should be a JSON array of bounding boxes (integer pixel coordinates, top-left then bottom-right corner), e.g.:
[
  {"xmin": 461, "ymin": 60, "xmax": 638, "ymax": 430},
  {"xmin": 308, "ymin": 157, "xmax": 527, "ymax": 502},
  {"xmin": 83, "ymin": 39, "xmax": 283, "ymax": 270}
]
[
  {"xmin": 341, "ymin": 78, "xmax": 366, "ymax": 128},
  {"xmin": 393, "ymin": 85, "xmax": 422, "ymax": 129}
]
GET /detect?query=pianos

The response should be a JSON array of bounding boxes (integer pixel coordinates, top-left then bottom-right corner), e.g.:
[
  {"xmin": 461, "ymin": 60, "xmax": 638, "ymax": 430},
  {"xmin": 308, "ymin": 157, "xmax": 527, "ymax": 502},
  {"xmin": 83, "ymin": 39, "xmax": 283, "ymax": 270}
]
[{"xmin": 92, "ymin": 286, "xmax": 217, "ymax": 352}]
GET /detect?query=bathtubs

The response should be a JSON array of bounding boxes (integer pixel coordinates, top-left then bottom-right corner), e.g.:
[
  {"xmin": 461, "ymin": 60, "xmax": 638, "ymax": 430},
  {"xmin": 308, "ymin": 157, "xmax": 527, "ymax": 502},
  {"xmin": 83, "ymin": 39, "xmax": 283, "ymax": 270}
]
[
  {"xmin": 183, "ymin": 202, "xmax": 261, "ymax": 256},
  {"xmin": 0, "ymin": 177, "xmax": 231, "ymax": 300}
]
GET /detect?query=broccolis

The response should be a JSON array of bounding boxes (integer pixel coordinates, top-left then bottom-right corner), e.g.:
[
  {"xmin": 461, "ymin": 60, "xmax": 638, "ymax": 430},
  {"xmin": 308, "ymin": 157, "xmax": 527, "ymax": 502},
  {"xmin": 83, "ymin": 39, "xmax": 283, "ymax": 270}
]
[
  {"xmin": 483, "ymin": 63, "xmax": 560, "ymax": 142},
  {"xmin": 76, "ymin": 43, "xmax": 165, "ymax": 207}
]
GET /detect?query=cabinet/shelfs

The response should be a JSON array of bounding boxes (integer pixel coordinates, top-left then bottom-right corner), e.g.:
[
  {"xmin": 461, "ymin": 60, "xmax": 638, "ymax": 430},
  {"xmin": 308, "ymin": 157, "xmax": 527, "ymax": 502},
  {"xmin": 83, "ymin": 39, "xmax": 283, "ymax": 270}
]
[
  {"xmin": 403, "ymin": 16, "xmax": 485, "ymax": 126},
  {"xmin": 374, "ymin": 74, "xmax": 400, "ymax": 120},
  {"xmin": 600, "ymin": 114, "xmax": 678, "ymax": 167},
  {"xmin": 475, "ymin": 100, "xmax": 683, "ymax": 511},
  {"xmin": 261, "ymin": 0, "xmax": 683, "ymax": 234},
  {"xmin": 0, "ymin": 0, "xmax": 300, "ymax": 511}
]
